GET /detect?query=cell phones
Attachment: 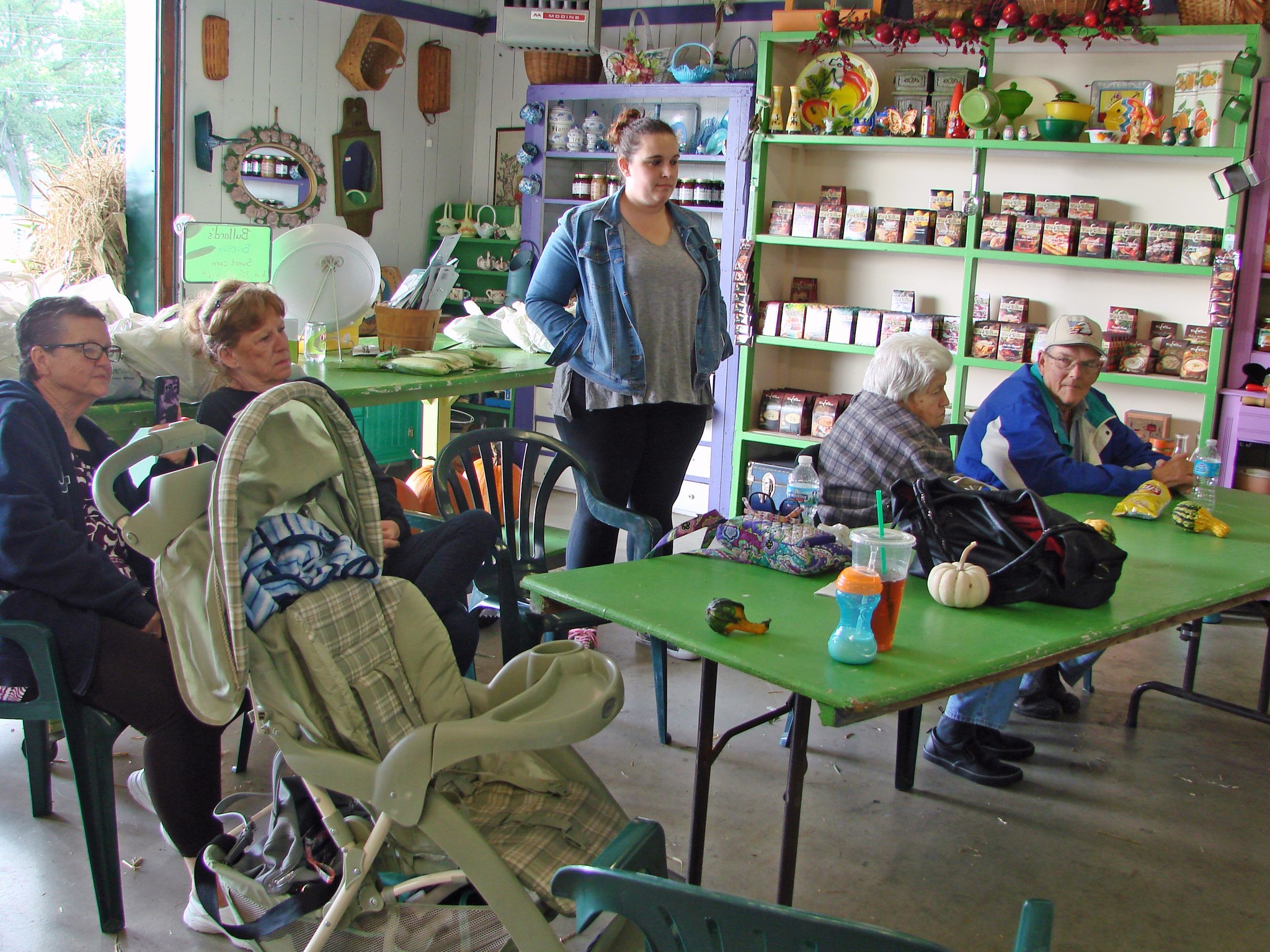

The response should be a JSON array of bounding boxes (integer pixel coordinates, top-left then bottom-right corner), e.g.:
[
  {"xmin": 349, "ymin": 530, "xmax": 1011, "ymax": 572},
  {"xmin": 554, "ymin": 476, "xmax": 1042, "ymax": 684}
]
[{"xmin": 154, "ymin": 375, "xmax": 180, "ymax": 425}]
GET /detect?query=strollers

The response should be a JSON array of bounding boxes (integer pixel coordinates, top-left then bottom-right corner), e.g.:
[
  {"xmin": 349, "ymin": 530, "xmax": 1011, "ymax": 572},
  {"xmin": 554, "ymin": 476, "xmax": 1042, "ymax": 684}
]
[{"xmin": 91, "ymin": 382, "xmax": 681, "ymax": 951}]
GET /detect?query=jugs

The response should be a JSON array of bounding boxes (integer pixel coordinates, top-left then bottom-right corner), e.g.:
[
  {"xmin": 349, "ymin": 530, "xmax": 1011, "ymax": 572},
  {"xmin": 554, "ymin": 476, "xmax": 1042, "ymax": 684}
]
[{"xmin": 1160, "ymin": 126, "xmax": 1193, "ymax": 146}]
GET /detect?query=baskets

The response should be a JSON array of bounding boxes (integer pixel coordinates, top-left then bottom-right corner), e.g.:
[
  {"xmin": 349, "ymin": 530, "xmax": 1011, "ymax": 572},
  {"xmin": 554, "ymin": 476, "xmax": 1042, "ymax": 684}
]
[
  {"xmin": 600, "ymin": 9, "xmax": 689, "ymax": 84},
  {"xmin": 1017, "ymin": 1, "xmax": 1106, "ymax": 25},
  {"xmin": 717, "ymin": 36, "xmax": 758, "ymax": 83},
  {"xmin": 524, "ymin": 51, "xmax": 602, "ymax": 85},
  {"xmin": 335, "ymin": 13, "xmax": 405, "ymax": 91},
  {"xmin": 1178, "ymin": 0, "xmax": 1242, "ymax": 26},
  {"xmin": 667, "ymin": 43, "xmax": 716, "ymax": 84},
  {"xmin": 912, "ymin": 0, "xmax": 1006, "ymax": 23},
  {"xmin": 418, "ymin": 38, "xmax": 451, "ymax": 125}
]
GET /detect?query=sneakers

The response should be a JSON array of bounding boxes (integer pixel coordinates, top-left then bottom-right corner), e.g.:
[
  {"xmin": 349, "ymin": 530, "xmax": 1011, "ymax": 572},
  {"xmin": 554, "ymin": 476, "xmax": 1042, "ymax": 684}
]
[
  {"xmin": 636, "ymin": 631, "xmax": 701, "ymax": 660},
  {"xmin": 183, "ymin": 891, "xmax": 253, "ymax": 951},
  {"xmin": 569, "ymin": 627, "xmax": 599, "ymax": 651},
  {"xmin": 128, "ymin": 768, "xmax": 177, "ymax": 850}
]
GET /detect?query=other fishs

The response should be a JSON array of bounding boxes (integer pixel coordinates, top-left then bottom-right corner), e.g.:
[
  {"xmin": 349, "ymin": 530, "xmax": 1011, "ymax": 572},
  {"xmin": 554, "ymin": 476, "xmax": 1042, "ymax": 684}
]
[{"xmin": 1104, "ymin": 98, "xmax": 1166, "ymax": 144}]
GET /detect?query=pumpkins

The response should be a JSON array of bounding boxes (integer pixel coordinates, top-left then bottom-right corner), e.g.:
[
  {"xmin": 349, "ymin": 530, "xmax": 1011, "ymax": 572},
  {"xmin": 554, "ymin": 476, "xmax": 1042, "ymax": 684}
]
[
  {"xmin": 927, "ymin": 540, "xmax": 989, "ymax": 608},
  {"xmin": 1171, "ymin": 501, "xmax": 1230, "ymax": 537},
  {"xmin": 393, "ymin": 478, "xmax": 423, "ymax": 535},
  {"xmin": 406, "ymin": 448, "xmax": 474, "ymax": 518},
  {"xmin": 462, "ymin": 446, "xmax": 523, "ymax": 526},
  {"xmin": 704, "ymin": 597, "xmax": 771, "ymax": 635},
  {"xmin": 1083, "ymin": 518, "xmax": 1117, "ymax": 545}
]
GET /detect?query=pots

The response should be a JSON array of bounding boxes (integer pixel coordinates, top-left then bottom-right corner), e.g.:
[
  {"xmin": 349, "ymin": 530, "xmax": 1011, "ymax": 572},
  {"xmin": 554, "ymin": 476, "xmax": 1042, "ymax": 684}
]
[{"xmin": 958, "ymin": 55, "xmax": 1001, "ymax": 131}]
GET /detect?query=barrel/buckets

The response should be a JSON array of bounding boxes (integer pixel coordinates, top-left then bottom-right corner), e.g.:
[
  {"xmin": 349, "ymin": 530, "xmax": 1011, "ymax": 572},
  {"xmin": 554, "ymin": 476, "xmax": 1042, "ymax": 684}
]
[
  {"xmin": 1233, "ymin": 464, "xmax": 1270, "ymax": 495},
  {"xmin": 373, "ymin": 303, "xmax": 443, "ymax": 353}
]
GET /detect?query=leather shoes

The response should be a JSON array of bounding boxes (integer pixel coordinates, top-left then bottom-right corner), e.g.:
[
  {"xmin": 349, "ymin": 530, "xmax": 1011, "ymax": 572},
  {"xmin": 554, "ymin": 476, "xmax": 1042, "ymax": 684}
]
[
  {"xmin": 938, "ymin": 705, "xmax": 1034, "ymax": 758},
  {"xmin": 922, "ymin": 726, "xmax": 1022, "ymax": 785}
]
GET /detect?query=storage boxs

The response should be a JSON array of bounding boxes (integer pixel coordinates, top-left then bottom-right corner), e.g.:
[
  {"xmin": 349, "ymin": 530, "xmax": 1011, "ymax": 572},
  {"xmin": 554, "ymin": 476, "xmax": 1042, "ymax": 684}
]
[{"xmin": 756, "ymin": 61, "xmax": 1241, "ymax": 446}]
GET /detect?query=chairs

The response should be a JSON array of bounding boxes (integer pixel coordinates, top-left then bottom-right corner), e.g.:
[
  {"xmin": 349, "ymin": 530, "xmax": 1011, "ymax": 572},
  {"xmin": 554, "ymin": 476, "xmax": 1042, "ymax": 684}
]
[
  {"xmin": 433, "ymin": 427, "xmax": 671, "ymax": 745},
  {"xmin": 1, "ymin": 619, "xmax": 255, "ymax": 936},
  {"xmin": 548, "ymin": 817, "xmax": 1056, "ymax": 952}
]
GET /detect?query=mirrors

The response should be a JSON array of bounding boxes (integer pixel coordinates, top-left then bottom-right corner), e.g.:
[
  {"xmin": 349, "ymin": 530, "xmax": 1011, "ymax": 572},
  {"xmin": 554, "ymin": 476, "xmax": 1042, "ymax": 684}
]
[
  {"xmin": 240, "ymin": 143, "xmax": 317, "ymax": 213},
  {"xmin": 333, "ymin": 98, "xmax": 383, "ymax": 236}
]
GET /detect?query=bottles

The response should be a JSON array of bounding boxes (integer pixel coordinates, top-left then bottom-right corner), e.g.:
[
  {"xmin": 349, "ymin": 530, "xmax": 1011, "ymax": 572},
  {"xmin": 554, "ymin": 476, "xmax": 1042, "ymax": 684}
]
[
  {"xmin": 1190, "ymin": 438, "xmax": 1221, "ymax": 513},
  {"xmin": 1189, "ymin": 434, "xmax": 1199, "ymax": 463},
  {"xmin": 827, "ymin": 546, "xmax": 883, "ymax": 665},
  {"xmin": 786, "ymin": 455, "xmax": 820, "ymax": 527},
  {"xmin": 1170, "ymin": 432, "xmax": 1190, "ymax": 460},
  {"xmin": 919, "ymin": 105, "xmax": 935, "ymax": 137},
  {"xmin": 1002, "ymin": 125, "xmax": 1028, "ymax": 140},
  {"xmin": 768, "ymin": 85, "xmax": 803, "ymax": 134}
]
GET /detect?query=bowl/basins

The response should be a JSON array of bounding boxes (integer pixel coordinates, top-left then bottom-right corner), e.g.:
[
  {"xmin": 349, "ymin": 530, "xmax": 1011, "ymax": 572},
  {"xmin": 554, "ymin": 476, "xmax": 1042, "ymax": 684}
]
[
  {"xmin": 1034, "ymin": 102, "xmax": 1095, "ymax": 142},
  {"xmin": 1084, "ymin": 130, "xmax": 1128, "ymax": 144}
]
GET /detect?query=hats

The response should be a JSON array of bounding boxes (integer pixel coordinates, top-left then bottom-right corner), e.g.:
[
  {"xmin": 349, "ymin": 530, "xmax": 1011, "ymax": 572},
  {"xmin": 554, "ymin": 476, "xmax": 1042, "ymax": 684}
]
[{"xmin": 1045, "ymin": 314, "xmax": 1108, "ymax": 357}]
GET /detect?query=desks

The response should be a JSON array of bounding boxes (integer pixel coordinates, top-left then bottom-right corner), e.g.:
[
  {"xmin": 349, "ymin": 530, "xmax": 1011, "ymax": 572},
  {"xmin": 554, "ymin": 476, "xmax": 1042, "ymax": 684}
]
[
  {"xmin": 85, "ymin": 333, "xmax": 555, "ymax": 453},
  {"xmin": 520, "ymin": 486, "xmax": 1270, "ymax": 906}
]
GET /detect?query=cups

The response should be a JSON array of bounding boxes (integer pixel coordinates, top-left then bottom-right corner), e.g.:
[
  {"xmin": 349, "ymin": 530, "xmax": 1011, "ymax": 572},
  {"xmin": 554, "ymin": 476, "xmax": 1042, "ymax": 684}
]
[
  {"xmin": 450, "ymin": 288, "xmax": 471, "ymax": 301},
  {"xmin": 304, "ymin": 321, "xmax": 326, "ymax": 363},
  {"xmin": 848, "ymin": 526, "xmax": 916, "ymax": 652},
  {"xmin": 1222, "ymin": 46, "xmax": 1261, "ymax": 124},
  {"xmin": 516, "ymin": 101, "xmax": 543, "ymax": 196},
  {"xmin": 486, "ymin": 289, "xmax": 506, "ymax": 305}
]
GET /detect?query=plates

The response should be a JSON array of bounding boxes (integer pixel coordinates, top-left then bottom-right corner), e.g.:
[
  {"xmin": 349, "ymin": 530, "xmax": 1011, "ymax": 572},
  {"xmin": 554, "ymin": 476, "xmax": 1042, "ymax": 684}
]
[
  {"xmin": 688, "ymin": 117, "xmax": 726, "ymax": 155},
  {"xmin": 794, "ymin": 51, "xmax": 880, "ymax": 135},
  {"xmin": 992, "ymin": 75, "xmax": 1058, "ymax": 141}
]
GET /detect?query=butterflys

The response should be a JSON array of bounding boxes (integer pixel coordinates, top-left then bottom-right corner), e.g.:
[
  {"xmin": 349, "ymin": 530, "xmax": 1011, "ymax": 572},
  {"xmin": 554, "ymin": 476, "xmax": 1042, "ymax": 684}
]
[{"xmin": 887, "ymin": 108, "xmax": 918, "ymax": 135}]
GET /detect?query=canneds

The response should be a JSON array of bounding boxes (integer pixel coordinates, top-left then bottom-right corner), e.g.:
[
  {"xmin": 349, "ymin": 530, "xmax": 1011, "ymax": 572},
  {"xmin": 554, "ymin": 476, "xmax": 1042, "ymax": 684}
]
[
  {"xmin": 240, "ymin": 154, "xmax": 303, "ymax": 180},
  {"xmin": 1254, "ymin": 318, "xmax": 1270, "ymax": 353},
  {"xmin": 571, "ymin": 173, "xmax": 724, "ymax": 208}
]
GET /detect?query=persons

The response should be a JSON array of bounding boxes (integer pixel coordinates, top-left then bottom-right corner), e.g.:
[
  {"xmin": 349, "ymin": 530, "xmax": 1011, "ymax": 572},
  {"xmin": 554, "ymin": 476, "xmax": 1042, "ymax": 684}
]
[
  {"xmin": 816, "ymin": 333, "xmax": 1035, "ymax": 784},
  {"xmin": 184, "ymin": 279, "xmax": 496, "ymax": 674},
  {"xmin": 0, "ymin": 297, "xmax": 262, "ymax": 951},
  {"xmin": 953, "ymin": 314, "xmax": 1197, "ymax": 720},
  {"xmin": 525, "ymin": 107, "xmax": 732, "ymax": 660}
]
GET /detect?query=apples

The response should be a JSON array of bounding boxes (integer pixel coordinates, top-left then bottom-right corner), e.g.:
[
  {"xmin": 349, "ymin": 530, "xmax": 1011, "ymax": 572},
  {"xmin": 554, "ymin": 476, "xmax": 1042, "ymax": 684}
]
[{"xmin": 822, "ymin": 0, "xmax": 1143, "ymax": 43}]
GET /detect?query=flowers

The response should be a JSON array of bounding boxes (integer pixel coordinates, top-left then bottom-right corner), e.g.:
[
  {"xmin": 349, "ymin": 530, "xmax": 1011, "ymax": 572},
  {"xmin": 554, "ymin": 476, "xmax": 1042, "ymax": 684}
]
[
  {"xmin": 221, "ymin": 126, "xmax": 328, "ymax": 229},
  {"xmin": 609, "ymin": 29, "xmax": 660, "ymax": 85}
]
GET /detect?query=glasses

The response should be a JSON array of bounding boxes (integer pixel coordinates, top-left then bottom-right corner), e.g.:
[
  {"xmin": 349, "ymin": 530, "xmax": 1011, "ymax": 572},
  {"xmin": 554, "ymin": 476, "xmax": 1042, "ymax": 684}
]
[
  {"xmin": 41, "ymin": 342, "xmax": 122, "ymax": 362},
  {"xmin": 1046, "ymin": 352, "xmax": 1102, "ymax": 374},
  {"xmin": 741, "ymin": 492, "xmax": 804, "ymax": 524}
]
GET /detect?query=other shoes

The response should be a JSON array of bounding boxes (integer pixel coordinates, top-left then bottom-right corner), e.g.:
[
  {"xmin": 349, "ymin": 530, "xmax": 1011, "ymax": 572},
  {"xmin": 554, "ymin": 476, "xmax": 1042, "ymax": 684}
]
[
  {"xmin": 1014, "ymin": 689, "xmax": 1062, "ymax": 718},
  {"xmin": 1050, "ymin": 686, "xmax": 1081, "ymax": 712}
]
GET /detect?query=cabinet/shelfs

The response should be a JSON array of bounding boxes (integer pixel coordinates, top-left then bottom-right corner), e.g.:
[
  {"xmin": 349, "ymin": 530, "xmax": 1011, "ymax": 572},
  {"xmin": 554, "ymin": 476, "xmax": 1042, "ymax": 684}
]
[
  {"xmin": 1216, "ymin": 78, "xmax": 1270, "ymax": 489},
  {"xmin": 720, "ymin": 24, "xmax": 1269, "ymax": 508},
  {"xmin": 512, "ymin": 81, "xmax": 754, "ymax": 517},
  {"xmin": 425, "ymin": 203, "xmax": 522, "ymax": 314}
]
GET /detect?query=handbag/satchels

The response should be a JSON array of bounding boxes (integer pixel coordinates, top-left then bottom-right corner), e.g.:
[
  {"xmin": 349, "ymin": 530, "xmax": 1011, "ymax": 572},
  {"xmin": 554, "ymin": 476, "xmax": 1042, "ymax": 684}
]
[
  {"xmin": 889, "ymin": 476, "xmax": 1129, "ymax": 611},
  {"xmin": 212, "ymin": 748, "xmax": 368, "ymax": 894},
  {"xmin": 716, "ymin": 514, "xmax": 853, "ymax": 577}
]
